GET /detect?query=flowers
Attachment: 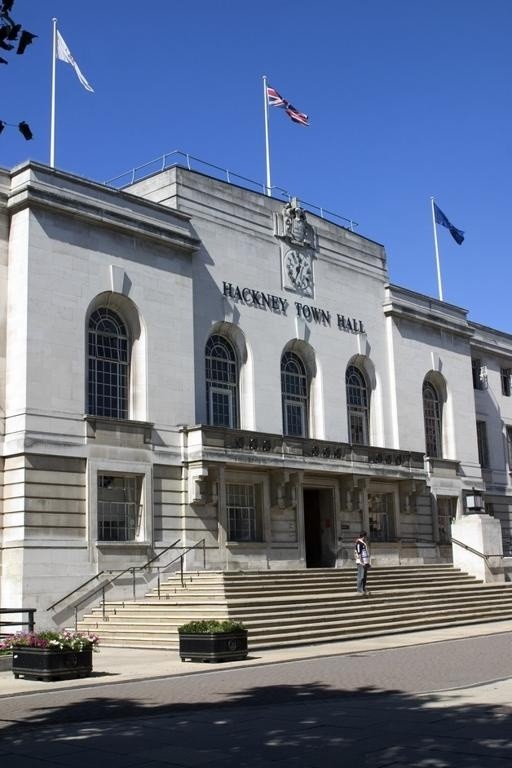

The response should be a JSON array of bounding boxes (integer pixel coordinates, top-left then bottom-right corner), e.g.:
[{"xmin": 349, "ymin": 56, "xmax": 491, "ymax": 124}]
[{"xmin": 0, "ymin": 627, "xmax": 101, "ymax": 656}]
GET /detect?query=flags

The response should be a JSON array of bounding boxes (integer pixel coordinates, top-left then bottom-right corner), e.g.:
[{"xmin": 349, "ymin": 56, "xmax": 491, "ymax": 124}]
[
  {"xmin": 430, "ymin": 194, "xmax": 467, "ymax": 246},
  {"xmin": 51, "ymin": 13, "xmax": 97, "ymax": 97},
  {"xmin": 262, "ymin": 72, "xmax": 311, "ymax": 130}
]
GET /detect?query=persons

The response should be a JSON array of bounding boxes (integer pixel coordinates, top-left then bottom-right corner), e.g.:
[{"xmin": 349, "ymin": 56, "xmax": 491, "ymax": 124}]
[{"xmin": 352, "ymin": 530, "xmax": 373, "ymax": 596}]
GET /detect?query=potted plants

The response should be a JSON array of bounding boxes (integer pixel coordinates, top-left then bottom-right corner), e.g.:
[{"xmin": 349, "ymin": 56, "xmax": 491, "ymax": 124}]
[{"xmin": 178, "ymin": 619, "xmax": 248, "ymax": 663}]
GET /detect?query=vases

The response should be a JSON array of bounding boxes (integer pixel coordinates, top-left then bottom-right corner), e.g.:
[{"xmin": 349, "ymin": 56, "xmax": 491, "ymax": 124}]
[{"xmin": 12, "ymin": 644, "xmax": 92, "ymax": 682}]
